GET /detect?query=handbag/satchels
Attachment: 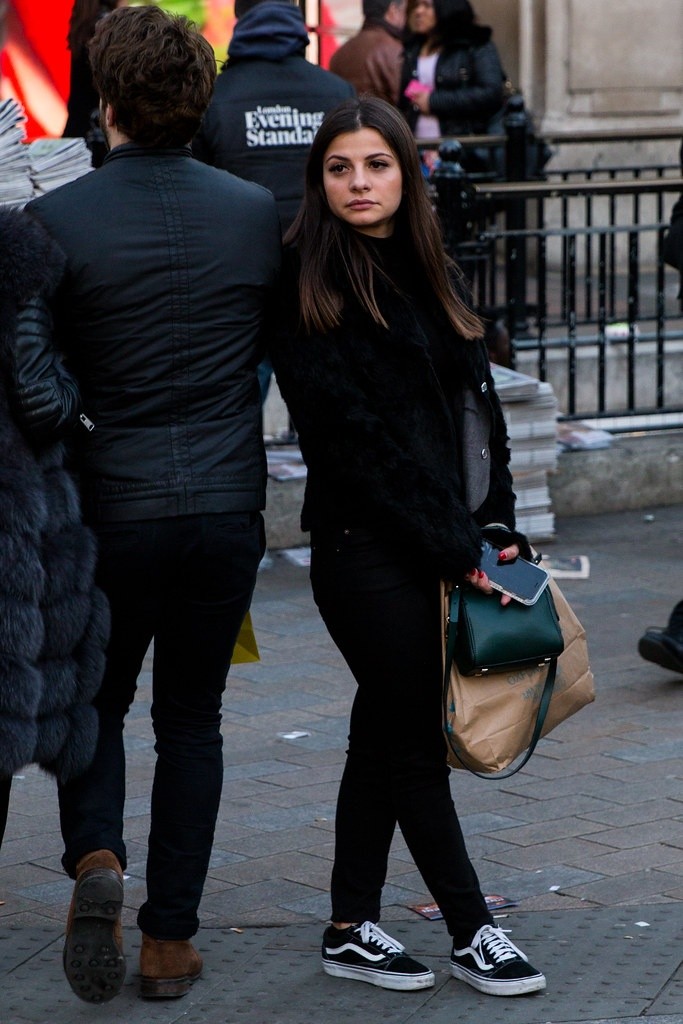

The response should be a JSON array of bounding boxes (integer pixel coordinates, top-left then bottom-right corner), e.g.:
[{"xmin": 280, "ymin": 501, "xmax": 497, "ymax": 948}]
[
  {"xmin": 502, "ymin": 91, "xmax": 552, "ymax": 184},
  {"xmin": 437, "ymin": 562, "xmax": 599, "ymax": 780}
]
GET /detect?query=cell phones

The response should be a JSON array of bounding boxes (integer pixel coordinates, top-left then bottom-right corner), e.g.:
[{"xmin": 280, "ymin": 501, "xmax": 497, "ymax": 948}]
[
  {"xmin": 476, "ymin": 539, "xmax": 550, "ymax": 606},
  {"xmin": 404, "ymin": 80, "xmax": 431, "ymax": 99}
]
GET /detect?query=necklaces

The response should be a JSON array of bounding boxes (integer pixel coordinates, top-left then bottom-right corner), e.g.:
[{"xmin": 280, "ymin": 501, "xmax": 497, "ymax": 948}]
[{"xmin": 371, "ymin": 260, "xmax": 403, "ymax": 295}]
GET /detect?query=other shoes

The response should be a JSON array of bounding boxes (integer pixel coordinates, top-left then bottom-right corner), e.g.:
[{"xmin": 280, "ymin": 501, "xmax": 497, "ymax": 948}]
[
  {"xmin": 139, "ymin": 933, "xmax": 203, "ymax": 999},
  {"xmin": 62, "ymin": 851, "xmax": 127, "ymax": 1004},
  {"xmin": 638, "ymin": 621, "xmax": 683, "ymax": 672}
]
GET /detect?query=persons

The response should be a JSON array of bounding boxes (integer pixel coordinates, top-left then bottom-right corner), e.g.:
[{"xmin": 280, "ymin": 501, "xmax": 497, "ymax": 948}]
[
  {"xmin": 637, "ymin": 142, "xmax": 683, "ymax": 676},
  {"xmin": 43, "ymin": 0, "xmax": 509, "ymax": 441},
  {"xmin": 24, "ymin": 5, "xmax": 281, "ymax": 1004},
  {"xmin": 274, "ymin": 97, "xmax": 547, "ymax": 997},
  {"xmin": 0, "ymin": 205, "xmax": 111, "ymax": 848}
]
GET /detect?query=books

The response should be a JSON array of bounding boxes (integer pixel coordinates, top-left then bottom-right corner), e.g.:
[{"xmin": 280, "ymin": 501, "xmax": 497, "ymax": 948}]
[
  {"xmin": 265, "ymin": 361, "xmax": 616, "ymax": 578},
  {"xmin": 0, "ymin": 98, "xmax": 96, "ymax": 205}
]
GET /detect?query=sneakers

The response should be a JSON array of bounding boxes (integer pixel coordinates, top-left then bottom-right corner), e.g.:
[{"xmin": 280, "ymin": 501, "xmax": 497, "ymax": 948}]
[
  {"xmin": 322, "ymin": 919, "xmax": 435, "ymax": 991},
  {"xmin": 448, "ymin": 918, "xmax": 548, "ymax": 996}
]
